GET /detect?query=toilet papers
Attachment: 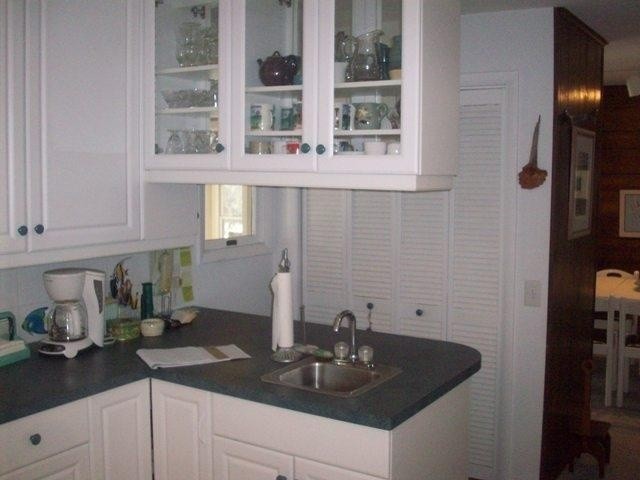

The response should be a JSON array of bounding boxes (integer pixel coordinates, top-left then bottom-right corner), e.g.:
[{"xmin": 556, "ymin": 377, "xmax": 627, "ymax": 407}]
[{"xmin": 268, "ymin": 272, "xmax": 295, "ymax": 351}]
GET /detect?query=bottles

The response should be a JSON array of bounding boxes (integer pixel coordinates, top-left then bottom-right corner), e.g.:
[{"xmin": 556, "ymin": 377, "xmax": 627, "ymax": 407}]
[{"xmin": 388, "ymin": 31, "xmax": 402, "ymax": 79}]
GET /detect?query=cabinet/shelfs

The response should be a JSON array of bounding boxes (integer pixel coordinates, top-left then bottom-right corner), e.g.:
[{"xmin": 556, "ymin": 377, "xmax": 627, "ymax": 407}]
[
  {"xmin": 2, "ymin": 378, "xmax": 152, "ymax": 479},
  {"xmin": 142, "ymin": 1, "xmax": 461, "ymax": 192},
  {"xmin": 0, "ymin": 0, "xmax": 141, "ymax": 255},
  {"xmin": 151, "ymin": 378, "xmax": 393, "ymax": 479}
]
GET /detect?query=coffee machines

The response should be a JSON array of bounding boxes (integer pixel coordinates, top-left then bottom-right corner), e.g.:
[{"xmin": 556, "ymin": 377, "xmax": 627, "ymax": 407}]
[{"xmin": 37, "ymin": 268, "xmax": 105, "ymax": 357}]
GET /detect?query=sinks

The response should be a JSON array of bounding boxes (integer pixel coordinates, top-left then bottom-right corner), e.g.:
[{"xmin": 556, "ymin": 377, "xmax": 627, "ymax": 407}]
[{"xmin": 260, "ymin": 355, "xmax": 403, "ymax": 400}]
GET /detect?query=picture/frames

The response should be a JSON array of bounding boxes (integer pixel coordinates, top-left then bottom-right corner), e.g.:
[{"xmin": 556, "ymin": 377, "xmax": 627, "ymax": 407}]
[
  {"xmin": 567, "ymin": 126, "xmax": 596, "ymax": 240},
  {"xmin": 619, "ymin": 189, "xmax": 640, "ymax": 238}
]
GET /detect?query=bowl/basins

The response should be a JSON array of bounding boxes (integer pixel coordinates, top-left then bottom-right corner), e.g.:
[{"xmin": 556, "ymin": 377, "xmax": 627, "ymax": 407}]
[
  {"xmin": 140, "ymin": 317, "xmax": 164, "ymax": 337},
  {"xmin": 363, "ymin": 140, "xmax": 400, "ymax": 156}
]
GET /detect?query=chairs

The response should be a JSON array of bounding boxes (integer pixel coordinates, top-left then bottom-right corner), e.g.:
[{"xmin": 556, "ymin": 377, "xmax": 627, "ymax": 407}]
[{"xmin": 592, "ymin": 268, "xmax": 640, "ymax": 408}]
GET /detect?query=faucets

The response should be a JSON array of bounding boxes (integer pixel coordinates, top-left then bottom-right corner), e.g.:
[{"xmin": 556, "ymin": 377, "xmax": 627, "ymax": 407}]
[{"xmin": 333, "ymin": 309, "xmax": 359, "ymax": 366}]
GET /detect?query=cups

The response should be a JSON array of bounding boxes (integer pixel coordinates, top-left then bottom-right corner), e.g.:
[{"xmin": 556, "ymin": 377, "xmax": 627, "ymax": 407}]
[{"xmin": 248, "ymin": 104, "xmax": 302, "ymax": 153}]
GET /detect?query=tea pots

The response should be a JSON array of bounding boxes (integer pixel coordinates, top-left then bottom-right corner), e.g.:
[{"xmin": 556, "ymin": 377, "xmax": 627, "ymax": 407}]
[
  {"xmin": 340, "ymin": 31, "xmax": 384, "ymax": 83},
  {"xmin": 255, "ymin": 50, "xmax": 297, "ymax": 86},
  {"xmin": 352, "ymin": 100, "xmax": 387, "ymax": 130}
]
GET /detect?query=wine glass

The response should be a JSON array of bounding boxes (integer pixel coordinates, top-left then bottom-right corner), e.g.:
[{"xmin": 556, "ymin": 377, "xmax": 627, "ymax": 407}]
[{"xmin": 167, "ymin": 127, "xmax": 211, "ymax": 153}]
